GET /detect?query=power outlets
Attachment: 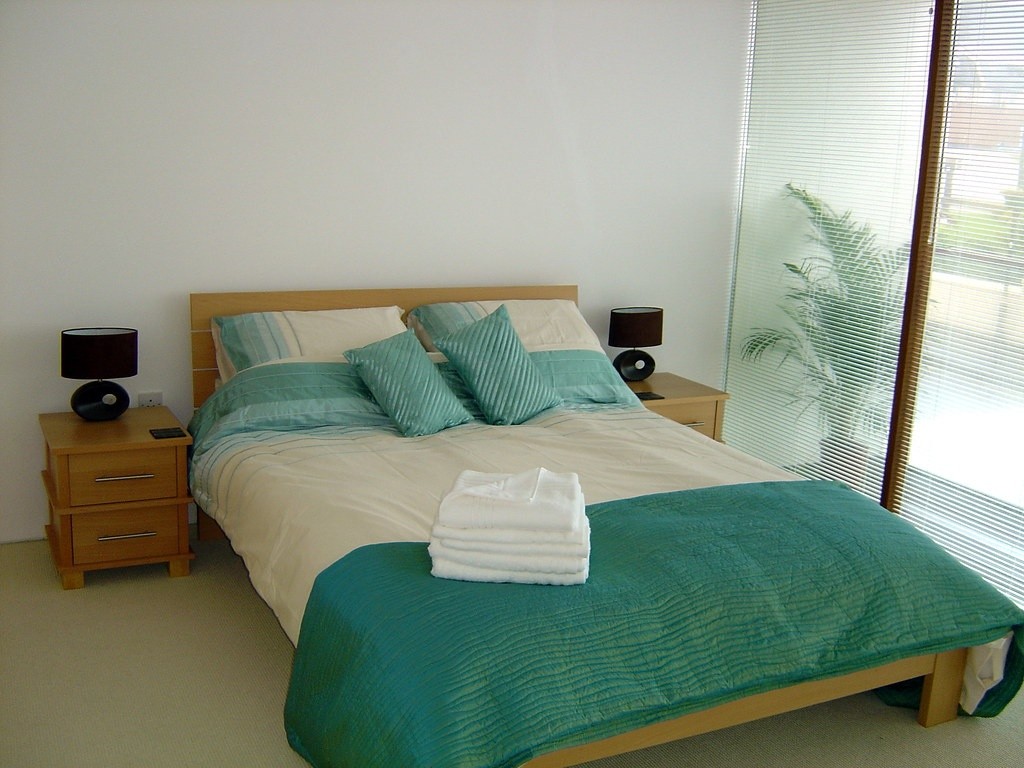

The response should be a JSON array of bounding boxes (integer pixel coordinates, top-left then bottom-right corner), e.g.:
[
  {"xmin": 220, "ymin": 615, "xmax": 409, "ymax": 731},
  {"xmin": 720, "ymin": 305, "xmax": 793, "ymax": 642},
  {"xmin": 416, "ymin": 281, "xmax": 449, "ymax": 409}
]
[{"xmin": 138, "ymin": 392, "xmax": 163, "ymax": 408}]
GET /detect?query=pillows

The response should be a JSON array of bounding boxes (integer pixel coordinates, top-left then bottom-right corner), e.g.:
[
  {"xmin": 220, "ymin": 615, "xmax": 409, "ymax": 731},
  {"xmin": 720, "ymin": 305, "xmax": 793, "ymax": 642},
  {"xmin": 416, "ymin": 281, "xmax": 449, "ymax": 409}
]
[
  {"xmin": 432, "ymin": 304, "xmax": 564, "ymax": 425},
  {"xmin": 407, "ymin": 299, "xmax": 601, "ymax": 346},
  {"xmin": 343, "ymin": 326, "xmax": 474, "ymax": 438},
  {"xmin": 211, "ymin": 305, "xmax": 407, "ymax": 384}
]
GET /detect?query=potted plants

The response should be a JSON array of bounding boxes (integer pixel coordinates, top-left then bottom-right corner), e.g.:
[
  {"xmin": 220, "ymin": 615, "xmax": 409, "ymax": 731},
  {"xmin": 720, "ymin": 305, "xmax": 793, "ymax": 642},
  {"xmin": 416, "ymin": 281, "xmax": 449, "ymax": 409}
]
[{"xmin": 740, "ymin": 182, "xmax": 911, "ymax": 483}]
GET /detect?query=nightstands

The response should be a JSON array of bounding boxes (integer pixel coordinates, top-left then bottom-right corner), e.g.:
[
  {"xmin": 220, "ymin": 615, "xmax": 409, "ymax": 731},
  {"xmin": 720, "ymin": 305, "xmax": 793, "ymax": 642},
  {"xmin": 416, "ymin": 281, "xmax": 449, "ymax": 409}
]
[
  {"xmin": 624, "ymin": 372, "xmax": 731, "ymax": 444},
  {"xmin": 39, "ymin": 405, "xmax": 196, "ymax": 590}
]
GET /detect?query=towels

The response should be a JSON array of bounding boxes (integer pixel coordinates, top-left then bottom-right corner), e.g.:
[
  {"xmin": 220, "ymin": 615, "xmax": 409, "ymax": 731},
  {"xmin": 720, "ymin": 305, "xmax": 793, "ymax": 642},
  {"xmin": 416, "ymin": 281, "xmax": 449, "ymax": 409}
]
[{"xmin": 428, "ymin": 467, "xmax": 590, "ymax": 585}]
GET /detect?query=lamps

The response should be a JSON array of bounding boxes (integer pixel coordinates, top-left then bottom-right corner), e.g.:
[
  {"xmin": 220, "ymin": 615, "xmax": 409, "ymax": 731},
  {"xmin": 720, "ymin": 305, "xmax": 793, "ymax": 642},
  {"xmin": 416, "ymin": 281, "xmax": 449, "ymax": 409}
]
[
  {"xmin": 608, "ymin": 308, "xmax": 663, "ymax": 381},
  {"xmin": 60, "ymin": 327, "xmax": 138, "ymax": 421}
]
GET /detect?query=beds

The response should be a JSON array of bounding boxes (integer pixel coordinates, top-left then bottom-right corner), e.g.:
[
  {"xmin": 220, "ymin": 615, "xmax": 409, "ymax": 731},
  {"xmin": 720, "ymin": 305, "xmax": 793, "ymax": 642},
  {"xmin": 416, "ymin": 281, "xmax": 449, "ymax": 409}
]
[{"xmin": 187, "ymin": 285, "xmax": 1024, "ymax": 768}]
